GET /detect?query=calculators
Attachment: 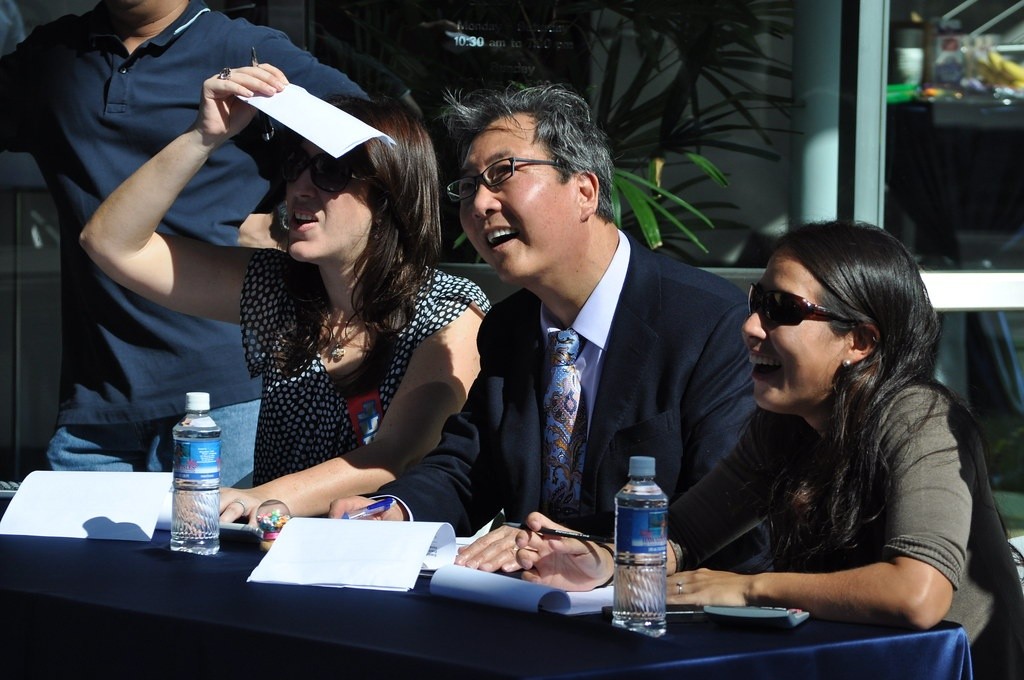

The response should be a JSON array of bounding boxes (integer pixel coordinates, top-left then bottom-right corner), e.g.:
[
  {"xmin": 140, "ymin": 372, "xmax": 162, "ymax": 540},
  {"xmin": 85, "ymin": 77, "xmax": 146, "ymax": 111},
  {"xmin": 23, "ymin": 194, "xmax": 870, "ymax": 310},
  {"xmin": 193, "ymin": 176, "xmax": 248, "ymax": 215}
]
[{"xmin": 703, "ymin": 603, "xmax": 810, "ymax": 631}]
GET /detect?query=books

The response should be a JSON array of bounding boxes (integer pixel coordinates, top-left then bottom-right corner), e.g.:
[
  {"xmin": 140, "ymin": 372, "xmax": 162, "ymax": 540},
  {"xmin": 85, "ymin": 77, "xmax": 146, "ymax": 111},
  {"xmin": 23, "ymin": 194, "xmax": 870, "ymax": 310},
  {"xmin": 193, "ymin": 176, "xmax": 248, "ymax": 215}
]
[
  {"xmin": 243, "ymin": 505, "xmax": 614, "ymax": 618},
  {"xmin": 1, "ymin": 469, "xmax": 174, "ymax": 542}
]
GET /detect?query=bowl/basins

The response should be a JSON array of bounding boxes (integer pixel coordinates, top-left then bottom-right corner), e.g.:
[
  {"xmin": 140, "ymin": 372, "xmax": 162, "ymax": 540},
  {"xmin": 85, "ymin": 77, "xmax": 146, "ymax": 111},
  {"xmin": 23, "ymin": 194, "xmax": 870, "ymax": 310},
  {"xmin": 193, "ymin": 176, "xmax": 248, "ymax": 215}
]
[{"xmin": 890, "ymin": 28, "xmax": 924, "ymax": 84}]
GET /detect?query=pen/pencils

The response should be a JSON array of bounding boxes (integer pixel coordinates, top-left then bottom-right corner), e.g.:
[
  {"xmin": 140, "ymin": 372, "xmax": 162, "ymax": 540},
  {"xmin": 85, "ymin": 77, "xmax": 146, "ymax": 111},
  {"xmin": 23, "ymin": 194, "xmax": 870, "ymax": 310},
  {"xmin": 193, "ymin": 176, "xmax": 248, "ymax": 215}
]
[
  {"xmin": 341, "ymin": 498, "xmax": 397, "ymax": 520},
  {"xmin": 502, "ymin": 521, "xmax": 591, "ymax": 540}
]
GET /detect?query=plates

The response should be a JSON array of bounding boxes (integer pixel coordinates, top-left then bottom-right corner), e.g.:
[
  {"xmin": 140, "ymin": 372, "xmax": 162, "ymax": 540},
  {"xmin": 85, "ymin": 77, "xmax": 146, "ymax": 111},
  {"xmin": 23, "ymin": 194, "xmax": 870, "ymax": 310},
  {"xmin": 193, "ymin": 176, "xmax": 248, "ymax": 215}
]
[{"xmin": 886, "ymin": 83, "xmax": 968, "ymax": 107}]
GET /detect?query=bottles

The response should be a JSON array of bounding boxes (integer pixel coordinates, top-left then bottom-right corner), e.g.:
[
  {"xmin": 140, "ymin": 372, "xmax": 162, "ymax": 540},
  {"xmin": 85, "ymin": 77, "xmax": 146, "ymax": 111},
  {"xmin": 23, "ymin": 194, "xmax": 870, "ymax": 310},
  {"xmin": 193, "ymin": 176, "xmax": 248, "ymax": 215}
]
[
  {"xmin": 170, "ymin": 391, "xmax": 221, "ymax": 555},
  {"xmin": 612, "ymin": 456, "xmax": 670, "ymax": 638}
]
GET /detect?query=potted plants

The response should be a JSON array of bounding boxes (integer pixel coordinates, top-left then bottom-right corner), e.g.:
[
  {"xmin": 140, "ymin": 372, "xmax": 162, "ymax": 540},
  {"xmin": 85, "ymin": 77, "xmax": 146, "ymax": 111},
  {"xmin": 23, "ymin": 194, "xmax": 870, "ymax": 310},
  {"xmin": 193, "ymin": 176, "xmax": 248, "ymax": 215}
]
[{"xmin": 319, "ymin": 0, "xmax": 800, "ymax": 264}]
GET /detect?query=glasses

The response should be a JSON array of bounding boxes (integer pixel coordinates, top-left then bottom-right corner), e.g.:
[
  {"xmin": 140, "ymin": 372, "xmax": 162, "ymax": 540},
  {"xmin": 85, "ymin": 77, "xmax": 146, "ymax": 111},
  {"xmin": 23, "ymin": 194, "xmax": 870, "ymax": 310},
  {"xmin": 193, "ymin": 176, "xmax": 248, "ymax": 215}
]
[
  {"xmin": 747, "ymin": 284, "xmax": 858, "ymax": 326},
  {"xmin": 279, "ymin": 147, "xmax": 370, "ymax": 192},
  {"xmin": 445, "ymin": 157, "xmax": 570, "ymax": 203}
]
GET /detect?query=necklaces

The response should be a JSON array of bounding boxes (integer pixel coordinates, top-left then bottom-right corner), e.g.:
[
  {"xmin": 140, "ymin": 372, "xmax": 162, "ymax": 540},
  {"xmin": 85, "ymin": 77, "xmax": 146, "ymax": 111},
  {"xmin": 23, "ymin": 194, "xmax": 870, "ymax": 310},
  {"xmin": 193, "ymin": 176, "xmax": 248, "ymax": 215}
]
[{"xmin": 322, "ymin": 314, "xmax": 361, "ymax": 360}]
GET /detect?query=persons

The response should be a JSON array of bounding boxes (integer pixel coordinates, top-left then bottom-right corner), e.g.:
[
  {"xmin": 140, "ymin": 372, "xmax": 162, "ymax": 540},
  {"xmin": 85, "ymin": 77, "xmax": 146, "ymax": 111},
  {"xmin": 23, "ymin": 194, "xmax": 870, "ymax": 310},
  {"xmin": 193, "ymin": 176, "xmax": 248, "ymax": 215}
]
[
  {"xmin": 515, "ymin": 221, "xmax": 1023, "ymax": 680},
  {"xmin": 933, "ymin": 39, "xmax": 965, "ymax": 88},
  {"xmin": 78, "ymin": 63, "xmax": 493, "ymax": 526},
  {"xmin": 326, "ymin": 83, "xmax": 774, "ymax": 574},
  {"xmin": 0, "ymin": 0, "xmax": 372, "ymax": 492}
]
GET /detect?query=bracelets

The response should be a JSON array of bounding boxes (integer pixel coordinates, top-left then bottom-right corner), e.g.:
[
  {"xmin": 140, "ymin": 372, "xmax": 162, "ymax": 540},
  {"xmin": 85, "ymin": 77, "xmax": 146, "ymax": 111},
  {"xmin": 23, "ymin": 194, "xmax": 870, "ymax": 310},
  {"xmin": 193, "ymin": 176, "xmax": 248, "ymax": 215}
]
[
  {"xmin": 667, "ymin": 538, "xmax": 684, "ymax": 573},
  {"xmin": 595, "ymin": 541, "xmax": 616, "ymax": 588}
]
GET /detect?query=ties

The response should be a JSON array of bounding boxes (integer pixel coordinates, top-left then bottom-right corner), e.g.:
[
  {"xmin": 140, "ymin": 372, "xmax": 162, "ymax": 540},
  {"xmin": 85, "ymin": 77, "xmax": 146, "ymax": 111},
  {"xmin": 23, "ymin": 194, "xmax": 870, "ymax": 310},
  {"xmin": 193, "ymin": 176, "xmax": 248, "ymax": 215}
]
[{"xmin": 541, "ymin": 329, "xmax": 587, "ymax": 527}]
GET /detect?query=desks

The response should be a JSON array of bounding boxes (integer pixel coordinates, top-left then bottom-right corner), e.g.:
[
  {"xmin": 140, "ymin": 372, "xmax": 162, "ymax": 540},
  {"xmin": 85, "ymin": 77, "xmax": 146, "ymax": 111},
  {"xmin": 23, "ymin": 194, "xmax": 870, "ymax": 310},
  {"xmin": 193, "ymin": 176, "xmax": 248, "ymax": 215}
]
[
  {"xmin": 435, "ymin": 262, "xmax": 1024, "ymax": 309},
  {"xmin": 0, "ymin": 531, "xmax": 974, "ymax": 680}
]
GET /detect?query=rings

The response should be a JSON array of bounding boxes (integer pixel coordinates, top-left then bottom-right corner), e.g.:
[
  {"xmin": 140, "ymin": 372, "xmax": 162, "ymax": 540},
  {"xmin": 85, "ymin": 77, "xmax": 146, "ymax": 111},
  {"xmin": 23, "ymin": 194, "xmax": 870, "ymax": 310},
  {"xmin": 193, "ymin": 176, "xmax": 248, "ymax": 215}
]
[
  {"xmin": 232, "ymin": 497, "xmax": 249, "ymax": 516},
  {"xmin": 219, "ymin": 67, "xmax": 232, "ymax": 80},
  {"xmin": 676, "ymin": 582, "xmax": 684, "ymax": 594},
  {"xmin": 513, "ymin": 547, "xmax": 518, "ymax": 551}
]
